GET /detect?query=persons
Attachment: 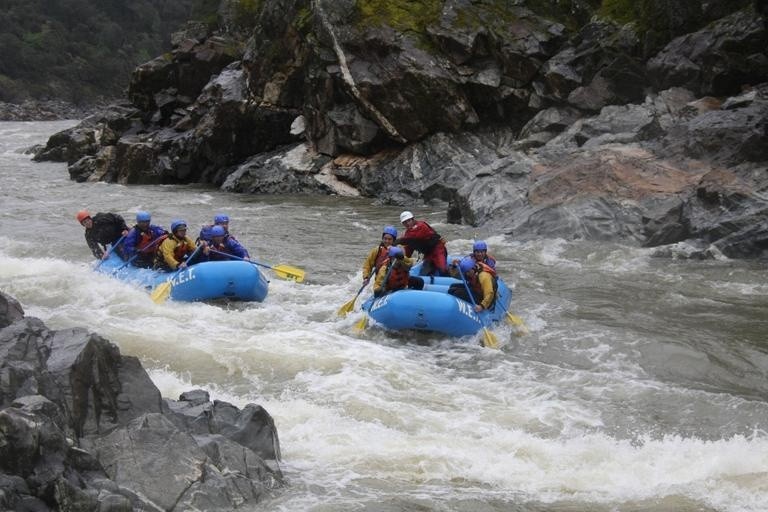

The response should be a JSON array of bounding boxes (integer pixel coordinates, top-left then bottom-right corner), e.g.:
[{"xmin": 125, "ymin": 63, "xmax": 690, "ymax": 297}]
[
  {"xmin": 395, "ymin": 211, "xmax": 448, "ymax": 276},
  {"xmin": 203, "ymin": 226, "xmax": 250, "ymax": 262},
  {"xmin": 374, "ymin": 245, "xmax": 425, "ymax": 298},
  {"xmin": 469, "ymin": 240, "xmax": 495, "ymax": 273},
  {"xmin": 123, "ymin": 212, "xmax": 167, "ymax": 269},
  {"xmin": 199, "ymin": 215, "xmax": 236, "ymax": 245},
  {"xmin": 152, "ymin": 219, "xmax": 208, "ymax": 273},
  {"xmin": 363, "ymin": 227, "xmax": 405, "ymax": 285},
  {"xmin": 448, "ymin": 259, "xmax": 498, "ymax": 313},
  {"xmin": 77, "ymin": 210, "xmax": 130, "ymax": 261}
]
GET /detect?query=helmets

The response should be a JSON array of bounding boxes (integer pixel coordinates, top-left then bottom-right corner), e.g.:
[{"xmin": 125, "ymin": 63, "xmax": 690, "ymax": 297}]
[
  {"xmin": 400, "ymin": 211, "xmax": 414, "ymax": 224},
  {"xmin": 459, "ymin": 259, "xmax": 474, "ymax": 274},
  {"xmin": 210, "ymin": 215, "xmax": 229, "ymax": 236},
  {"xmin": 137, "ymin": 212, "xmax": 151, "ymax": 222},
  {"xmin": 382, "ymin": 226, "xmax": 397, "ymax": 238},
  {"xmin": 171, "ymin": 219, "xmax": 186, "ymax": 231},
  {"xmin": 77, "ymin": 210, "xmax": 91, "ymax": 226},
  {"xmin": 473, "ymin": 240, "xmax": 487, "ymax": 252},
  {"xmin": 387, "ymin": 247, "xmax": 404, "ymax": 257}
]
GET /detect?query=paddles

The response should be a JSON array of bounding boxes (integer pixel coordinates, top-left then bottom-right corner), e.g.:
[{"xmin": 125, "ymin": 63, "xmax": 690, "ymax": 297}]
[
  {"xmin": 152, "ymin": 243, "xmax": 204, "ymax": 303},
  {"xmin": 209, "ymin": 250, "xmax": 304, "ymax": 282},
  {"xmin": 495, "ymin": 294, "xmax": 529, "ymax": 336},
  {"xmin": 354, "ymin": 259, "xmax": 397, "ymax": 331},
  {"xmin": 457, "ymin": 263, "xmax": 497, "ymax": 348},
  {"xmin": 338, "ymin": 268, "xmax": 375, "ymax": 318}
]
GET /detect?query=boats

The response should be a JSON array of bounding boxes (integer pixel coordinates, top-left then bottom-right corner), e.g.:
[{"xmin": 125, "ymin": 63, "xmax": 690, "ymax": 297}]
[
  {"xmin": 360, "ymin": 255, "xmax": 512, "ymax": 340},
  {"xmin": 94, "ymin": 245, "xmax": 271, "ymax": 304}
]
